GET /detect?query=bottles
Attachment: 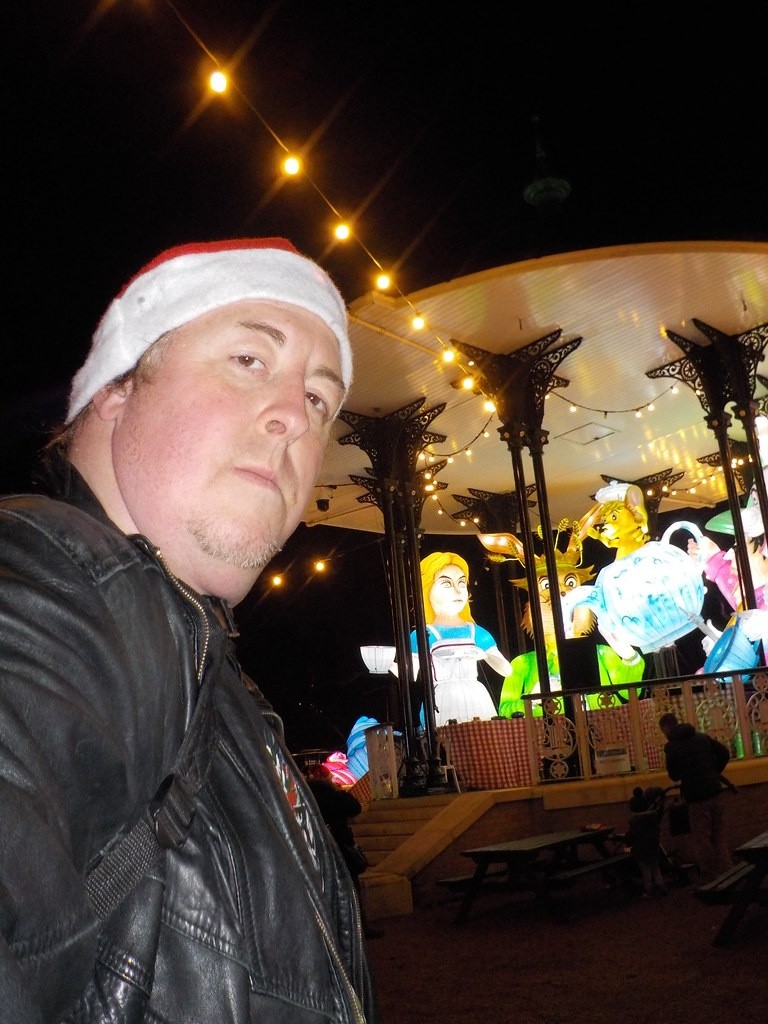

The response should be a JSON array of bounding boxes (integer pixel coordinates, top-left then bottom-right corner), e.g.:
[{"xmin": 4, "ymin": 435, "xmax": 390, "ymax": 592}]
[{"xmin": 641, "ymin": 755, "xmax": 649, "ymax": 774}]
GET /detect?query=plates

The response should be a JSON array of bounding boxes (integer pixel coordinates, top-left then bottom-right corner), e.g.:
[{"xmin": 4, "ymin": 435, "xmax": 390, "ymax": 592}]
[{"xmin": 471, "ymin": 720, "xmax": 482, "ymax": 721}]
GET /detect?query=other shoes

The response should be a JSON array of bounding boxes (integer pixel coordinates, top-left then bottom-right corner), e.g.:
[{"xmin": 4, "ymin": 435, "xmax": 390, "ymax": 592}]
[
  {"xmin": 656, "ymin": 890, "xmax": 669, "ymax": 896},
  {"xmin": 641, "ymin": 893, "xmax": 655, "ymax": 900}
]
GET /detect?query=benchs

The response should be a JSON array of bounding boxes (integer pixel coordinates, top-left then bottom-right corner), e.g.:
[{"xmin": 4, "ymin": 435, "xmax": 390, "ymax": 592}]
[
  {"xmin": 439, "ymin": 855, "xmax": 632, "ymax": 925},
  {"xmin": 701, "ymin": 860, "xmax": 768, "ymax": 948}
]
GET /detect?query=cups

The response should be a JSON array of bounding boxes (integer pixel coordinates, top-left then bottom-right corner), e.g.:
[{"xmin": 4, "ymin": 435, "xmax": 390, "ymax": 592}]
[
  {"xmin": 472, "ymin": 717, "xmax": 479, "ymax": 721},
  {"xmin": 448, "ymin": 719, "xmax": 458, "ymax": 725}
]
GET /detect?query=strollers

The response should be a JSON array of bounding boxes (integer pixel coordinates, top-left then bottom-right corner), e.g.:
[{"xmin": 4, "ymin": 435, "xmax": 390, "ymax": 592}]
[{"xmin": 608, "ymin": 784, "xmax": 683, "ymax": 858}]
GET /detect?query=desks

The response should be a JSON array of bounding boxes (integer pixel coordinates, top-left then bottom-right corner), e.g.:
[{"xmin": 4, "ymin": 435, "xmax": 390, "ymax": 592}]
[
  {"xmin": 461, "ymin": 826, "xmax": 617, "ymax": 880},
  {"xmin": 733, "ymin": 831, "xmax": 768, "ymax": 860}
]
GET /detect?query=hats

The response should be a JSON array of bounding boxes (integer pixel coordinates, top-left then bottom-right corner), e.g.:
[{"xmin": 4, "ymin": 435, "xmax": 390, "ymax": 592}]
[
  {"xmin": 629, "ymin": 786, "xmax": 652, "ymax": 811},
  {"xmin": 63, "ymin": 237, "xmax": 354, "ymax": 425}
]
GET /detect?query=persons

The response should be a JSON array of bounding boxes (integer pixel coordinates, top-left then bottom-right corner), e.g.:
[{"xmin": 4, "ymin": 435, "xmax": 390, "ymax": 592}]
[
  {"xmin": 659, "ymin": 714, "xmax": 730, "ymax": 875},
  {"xmin": 0, "ymin": 238, "xmax": 377, "ymax": 1024},
  {"xmin": 306, "ymin": 765, "xmax": 382, "ymax": 939},
  {"xmin": 625, "ymin": 787, "xmax": 666, "ymax": 898},
  {"xmin": 393, "ymin": 552, "xmax": 512, "ymax": 731},
  {"xmin": 687, "ymin": 535, "xmax": 768, "ymax": 674}
]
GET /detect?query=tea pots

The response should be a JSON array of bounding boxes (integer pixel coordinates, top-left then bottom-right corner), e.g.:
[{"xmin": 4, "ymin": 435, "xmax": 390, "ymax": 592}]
[{"xmin": 511, "ymin": 711, "xmax": 525, "ymax": 718}]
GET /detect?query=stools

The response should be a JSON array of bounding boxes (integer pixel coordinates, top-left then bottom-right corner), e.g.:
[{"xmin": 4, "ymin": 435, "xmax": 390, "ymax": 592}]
[{"xmin": 440, "ymin": 765, "xmax": 462, "ymax": 793}]
